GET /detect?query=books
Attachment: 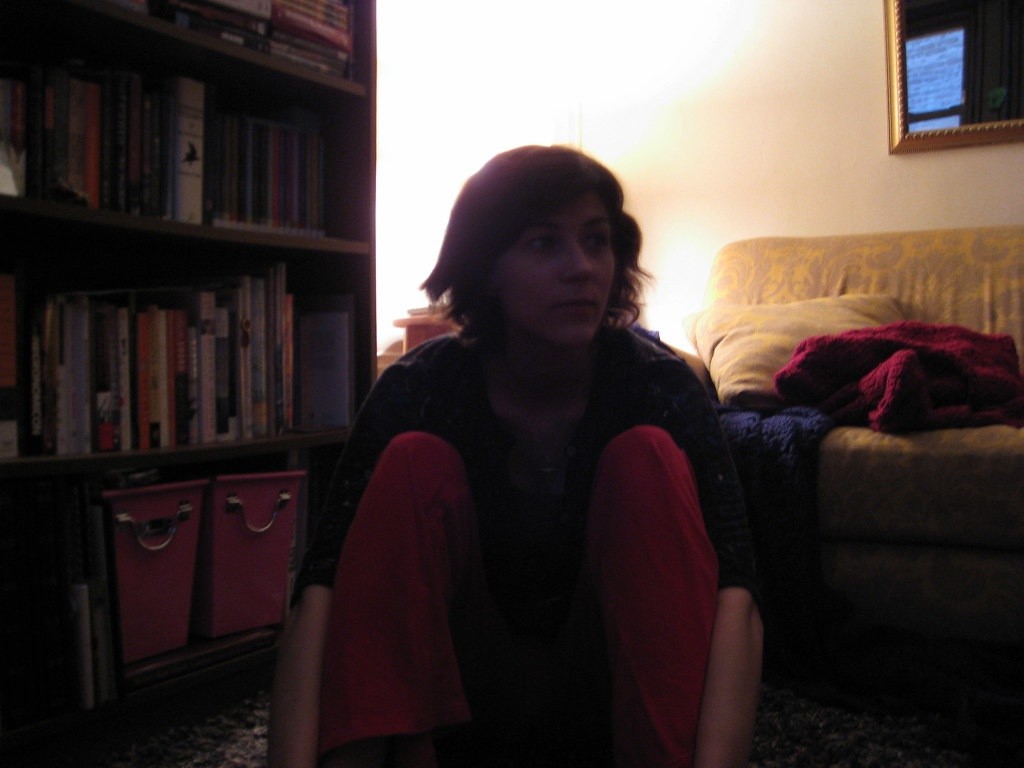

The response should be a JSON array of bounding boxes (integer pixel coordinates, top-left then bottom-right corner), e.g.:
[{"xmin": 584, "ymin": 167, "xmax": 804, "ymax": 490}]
[
  {"xmin": 3, "ymin": 2, "xmax": 358, "ymax": 462},
  {"xmin": 17, "ymin": 504, "xmax": 118, "ymax": 709}
]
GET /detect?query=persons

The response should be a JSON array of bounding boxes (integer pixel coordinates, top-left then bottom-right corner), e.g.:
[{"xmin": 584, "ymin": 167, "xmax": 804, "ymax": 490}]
[{"xmin": 271, "ymin": 144, "xmax": 769, "ymax": 768}]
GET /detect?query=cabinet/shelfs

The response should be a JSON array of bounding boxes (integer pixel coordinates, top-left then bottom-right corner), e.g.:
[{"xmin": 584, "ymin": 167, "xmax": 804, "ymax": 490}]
[{"xmin": 0, "ymin": 0, "xmax": 376, "ymax": 768}]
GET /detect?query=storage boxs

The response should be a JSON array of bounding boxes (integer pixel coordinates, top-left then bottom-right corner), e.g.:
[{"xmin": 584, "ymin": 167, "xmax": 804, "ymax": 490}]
[
  {"xmin": 194, "ymin": 472, "xmax": 308, "ymax": 638},
  {"xmin": 102, "ymin": 479, "xmax": 210, "ymax": 665}
]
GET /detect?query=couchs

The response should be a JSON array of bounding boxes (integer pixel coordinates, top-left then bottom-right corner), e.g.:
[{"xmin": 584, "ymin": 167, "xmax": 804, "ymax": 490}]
[{"xmin": 669, "ymin": 224, "xmax": 1023, "ymax": 646}]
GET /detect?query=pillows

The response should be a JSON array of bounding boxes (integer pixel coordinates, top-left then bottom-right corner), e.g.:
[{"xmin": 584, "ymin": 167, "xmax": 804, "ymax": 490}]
[{"xmin": 687, "ymin": 290, "xmax": 905, "ymax": 409}]
